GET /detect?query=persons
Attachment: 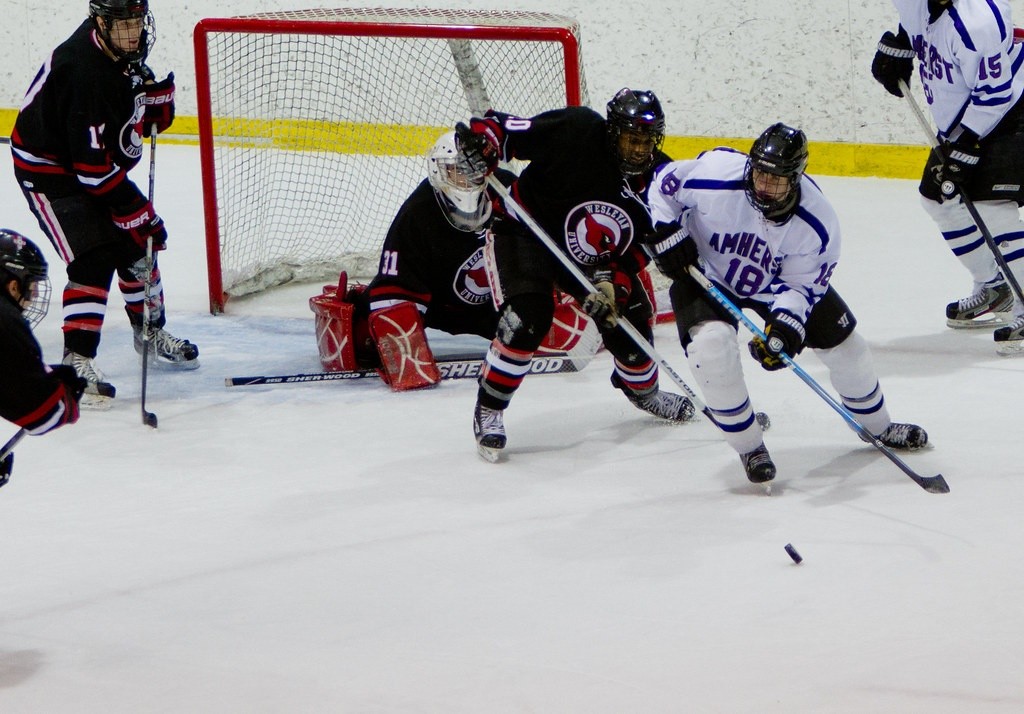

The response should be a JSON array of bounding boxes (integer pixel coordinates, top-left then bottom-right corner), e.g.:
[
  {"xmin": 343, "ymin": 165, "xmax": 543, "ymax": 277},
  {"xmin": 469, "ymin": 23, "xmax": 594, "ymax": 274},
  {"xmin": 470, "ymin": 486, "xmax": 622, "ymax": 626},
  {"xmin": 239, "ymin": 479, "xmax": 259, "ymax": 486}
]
[
  {"xmin": 354, "ymin": 129, "xmax": 678, "ymax": 392},
  {"xmin": 455, "ymin": 88, "xmax": 695, "ymax": 463},
  {"xmin": 648, "ymin": 123, "xmax": 934, "ymax": 495},
  {"xmin": 0, "ymin": 229, "xmax": 87, "ymax": 487},
  {"xmin": 8, "ymin": 0, "xmax": 199, "ymax": 411},
  {"xmin": 871, "ymin": 0, "xmax": 1024, "ymax": 356}
]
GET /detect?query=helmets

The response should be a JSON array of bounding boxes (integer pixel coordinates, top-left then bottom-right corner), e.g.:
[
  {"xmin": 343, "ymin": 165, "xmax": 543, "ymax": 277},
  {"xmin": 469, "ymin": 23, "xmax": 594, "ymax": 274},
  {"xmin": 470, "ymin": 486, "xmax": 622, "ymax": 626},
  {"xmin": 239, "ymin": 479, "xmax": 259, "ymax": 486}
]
[
  {"xmin": 89, "ymin": 0, "xmax": 156, "ymax": 75},
  {"xmin": 0, "ymin": 228, "xmax": 51, "ymax": 332},
  {"xmin": 742, "ymin": 123, "xmax": 809, "ymax": 214},
  {"xmin": 428, "ymin": 131, "xmax": 488, "ymax": 233},
  {"xmin": 606, "ymin": 87, "xmax": 668, "ymax": 177}
]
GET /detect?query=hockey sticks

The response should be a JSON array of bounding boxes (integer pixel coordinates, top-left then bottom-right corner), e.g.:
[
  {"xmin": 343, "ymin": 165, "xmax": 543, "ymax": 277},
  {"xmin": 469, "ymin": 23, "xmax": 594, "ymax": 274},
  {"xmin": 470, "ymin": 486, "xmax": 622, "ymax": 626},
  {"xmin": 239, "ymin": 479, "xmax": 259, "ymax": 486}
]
[
  {"xmin": 137, "ymin": 124, "xmax": 159, "ymax": 428},
  {"xmin": 685, "ymin": 264, "xmax": 957, "ymax": 495},
  {"xmin": 223, "ymin": 316, "xmax": 604, "ymax": 388},
  {"xmin": 485, "ymin": 173, "xmax": 771, "ymax": 433},
  {"xmin": 0, "ymin": 426, "xmax": 27, "ymax": 462},
  {"xmin": 898, "ymin": 78, "xmax": 1024, "ymax": 308}
]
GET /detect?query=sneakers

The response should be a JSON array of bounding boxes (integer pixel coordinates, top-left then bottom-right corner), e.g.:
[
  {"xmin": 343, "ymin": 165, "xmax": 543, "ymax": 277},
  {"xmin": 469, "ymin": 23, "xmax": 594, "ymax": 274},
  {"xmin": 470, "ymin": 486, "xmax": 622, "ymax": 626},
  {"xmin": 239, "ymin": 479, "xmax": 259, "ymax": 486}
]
[
  {"xmin": 621, "ymin": 387, "xmax": 696, "ymax": 421},
  {"xmin": 994, "ymin": 311, "xmax": 1024, "ymax": 355},
  {"xmin": 61, "ymin": 351, "xmax": 117, "ymax": 413},
  {"xmin": 133, "ymin": 327, "xmax": 200, "ymax": 374},
  {"xmin": 471, "ymin": 399, "xmax": 509, "ymax": 464},
  {"xmin": 944, "ymin": 273, "xmax": 1013, "ymax": 327},
  {"xmin": 739, "ymin": 439, "xmax": 777, "ymax": 496},
  {"xmin": 857, "ymin": 422, "xmax": 929, "ymax": 450}
]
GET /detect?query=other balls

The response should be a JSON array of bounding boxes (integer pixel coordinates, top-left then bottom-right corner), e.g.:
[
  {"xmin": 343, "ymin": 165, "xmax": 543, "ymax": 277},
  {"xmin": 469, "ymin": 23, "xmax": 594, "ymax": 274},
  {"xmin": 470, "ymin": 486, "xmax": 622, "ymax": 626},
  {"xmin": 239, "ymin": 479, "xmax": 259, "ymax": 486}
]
[{"xmin": 784, "ymin": 543, "xmax": 803, "ymax": 564}]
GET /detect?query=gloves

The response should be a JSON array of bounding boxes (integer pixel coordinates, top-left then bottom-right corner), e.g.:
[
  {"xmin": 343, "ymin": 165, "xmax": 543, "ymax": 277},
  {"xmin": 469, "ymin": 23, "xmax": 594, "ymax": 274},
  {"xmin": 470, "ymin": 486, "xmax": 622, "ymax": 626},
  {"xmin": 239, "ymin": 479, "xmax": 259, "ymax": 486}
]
[
  {"xmin": 128, "ymin": 216, "xmax": 169, "ymax": 251},
  {"xmin": 144, "ymin": 72, "xmax": 173, "ymax": 128},
  {"xmin": 870, "ymin": 31, "xmax": 913, "ymax": 98},
  {"xmin": 930, "ymin": 140, "xmax": 979, "ymax": 199},
  {"xmin": 650, "ymin": 221, "xmax": 699, "ymax": 281},
  {"xmin": 748, "ymin": 319, "xmax": 806, "ymax": 372},
  {"xmin": 583, "ymin": 283, "xmax": 619, "ymax": 328}
]
